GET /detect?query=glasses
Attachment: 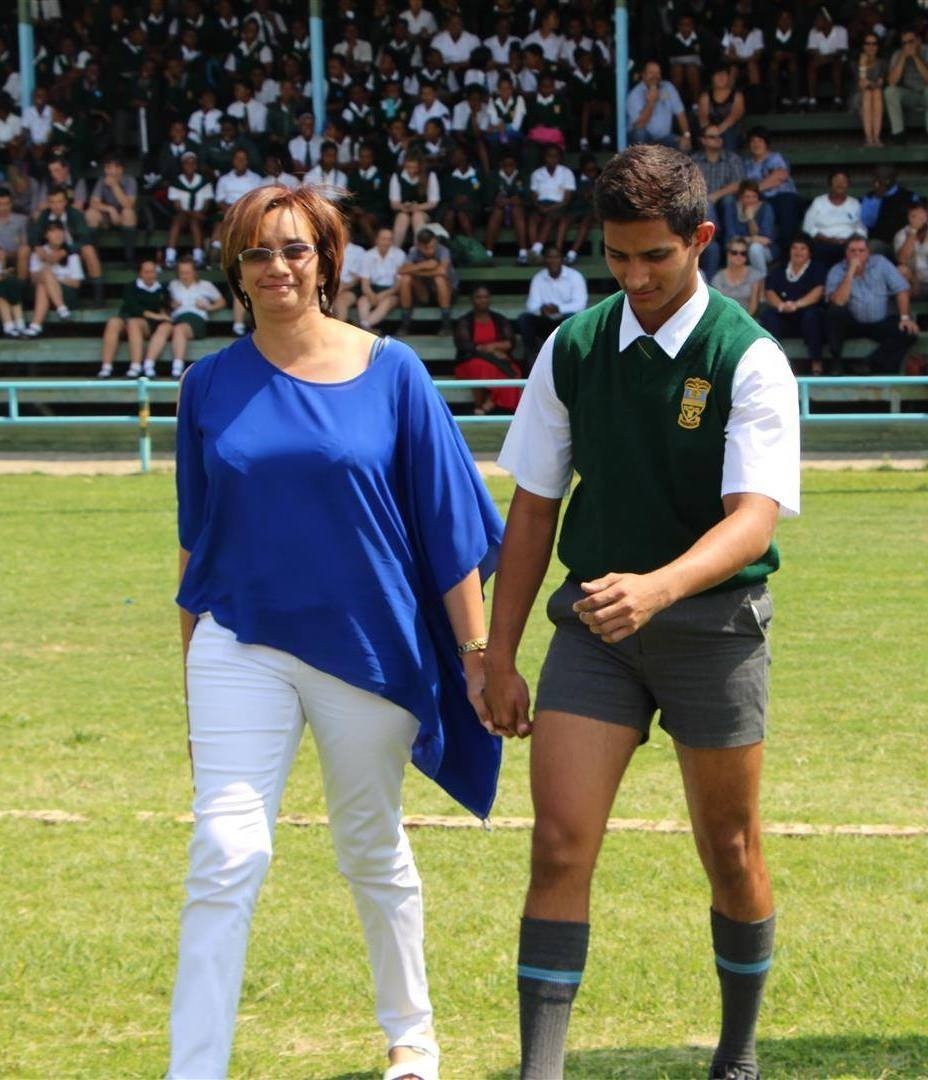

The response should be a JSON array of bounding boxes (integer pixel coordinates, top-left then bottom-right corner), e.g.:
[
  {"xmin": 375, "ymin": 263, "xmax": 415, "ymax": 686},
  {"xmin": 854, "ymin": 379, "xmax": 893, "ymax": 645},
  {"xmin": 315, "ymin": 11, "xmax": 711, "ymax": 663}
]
[
  {"xmin": 237, "ymin": 243, "xmax": 320, "ymax": 263},
  {"xmin": 847, "ymin": 246, "xmax": 868, "ymax": 253},
  {"xmin": 902, "ymin": 39, "xmax": 915, "ymax": 45},
  {"xmin": 864, "ymin": 41, "xmax": 878, "ymax": 45},
  {"xmin": 703, "ymin": 135, "xmax": 722, "ymax": 139},
  {"xmin": 728, "ymin": 248, "xmax": 748, "ymax": 256}
]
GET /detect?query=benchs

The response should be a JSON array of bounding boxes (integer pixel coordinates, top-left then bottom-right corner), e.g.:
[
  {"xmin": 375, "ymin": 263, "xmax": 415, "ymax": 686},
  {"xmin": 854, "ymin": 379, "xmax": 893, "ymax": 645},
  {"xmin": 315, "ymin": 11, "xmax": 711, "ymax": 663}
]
[{"xmin": 0, "ymin": 92, "xmax": 927, "ymax": 402}]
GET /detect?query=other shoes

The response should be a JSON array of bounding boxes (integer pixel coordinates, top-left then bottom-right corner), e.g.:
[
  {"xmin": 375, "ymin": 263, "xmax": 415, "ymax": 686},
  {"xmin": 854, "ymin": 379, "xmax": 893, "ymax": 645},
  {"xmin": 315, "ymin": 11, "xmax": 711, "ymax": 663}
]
[
  {"xmin": 16, "ymin": 325, "xmax": 27, "ymax": 335},
  {"xmin": 438, "ymin": 324, "xmax": 454, "ymax": 334},
  {"xmin": 126, "ymin": 367, "xmax": 142, "ymax": 378},
  {"xmin": 382, "ymin": 1034, "xmax": 440, "ymax": 1080},
  {"xmin": 98, "ymin": 368, "xmax": 114, "ymax": 379},
  {"xmin": 395, "ymin": 325, "xmax": 413, "ymax": 335},
  {"xmin": 142, "ymin": 365, "xmax": 157, "ymax": 378},
  {"xmin": 23, "ymin": 327, "xmax": 44, "ymax": 338},
  {"xmin": 57, "ymin": 306, "xmax": 71, "ymax": 319},
  {"xmin": 3, "ymin": 327, "xmax": 20, "ymax": 339},
  {"xmin": 172, "ymin": 366, "xmax": 185, "ymax": 381}
]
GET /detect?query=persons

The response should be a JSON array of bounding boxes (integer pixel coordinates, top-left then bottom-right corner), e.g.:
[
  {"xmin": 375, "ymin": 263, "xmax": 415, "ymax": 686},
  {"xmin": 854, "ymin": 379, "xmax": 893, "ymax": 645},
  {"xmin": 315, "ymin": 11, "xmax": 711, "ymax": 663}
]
[
  {"xmin": 0, "ymin": 0, "xmax": 616, "ymax": 415},
  {"xmin": 626, "ymin": 0, "xmax": 928, "ymax": 377},
  {"xmin": 482, "ymin": 145, "xmax": 800, "ymax": 1080},
  {"xmin": 167, "ymin": 183, "xmax": 505, "ymax": 1080}
]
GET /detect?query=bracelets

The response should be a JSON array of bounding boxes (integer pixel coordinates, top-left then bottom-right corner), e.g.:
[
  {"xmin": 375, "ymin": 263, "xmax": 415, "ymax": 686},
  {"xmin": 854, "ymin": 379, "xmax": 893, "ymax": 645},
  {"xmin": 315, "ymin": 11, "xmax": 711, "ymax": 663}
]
[
  {"xmin": 909, "ymin": 227, "xmax": 917, "ymax": 234},
  {"xmin": 859, "ymin": 67, "xmax": 866, "ymax": 71},
  {"xmin": 458, "ymin": 638, "xmax": 488, "ymax": 658},
  {"xmin": 900, "ymin": 315, "xmax": 910, "ymax": 320}
]
